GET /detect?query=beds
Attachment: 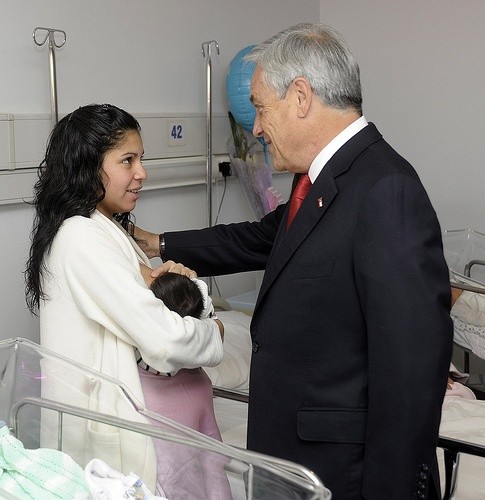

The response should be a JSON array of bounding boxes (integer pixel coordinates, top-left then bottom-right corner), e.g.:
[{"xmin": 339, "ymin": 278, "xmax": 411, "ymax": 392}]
[
  {"xmin": 0, "ymin": 338, "xmax": 332, "ymax": 500},
  {"xmin": 110, "ymin": 296, "xmax": 485, "ymax": 499}
]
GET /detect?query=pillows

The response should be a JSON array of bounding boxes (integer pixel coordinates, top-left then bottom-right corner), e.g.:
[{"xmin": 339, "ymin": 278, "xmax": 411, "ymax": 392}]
[{"xmin": 190, "ymin": 310, "xmax": 253, "ymax": 391}]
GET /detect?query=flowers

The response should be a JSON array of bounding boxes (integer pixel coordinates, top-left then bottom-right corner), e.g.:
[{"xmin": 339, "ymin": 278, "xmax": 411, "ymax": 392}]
[{"xmin": 227, "ymin": 111, "xmax": 285, "ymax": 220}]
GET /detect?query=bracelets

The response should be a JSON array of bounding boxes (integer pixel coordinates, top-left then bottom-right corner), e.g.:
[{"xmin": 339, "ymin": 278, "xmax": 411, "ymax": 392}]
[{"xmin": 157, "ymin": 233, "xmax": 166, "ymax": 261}]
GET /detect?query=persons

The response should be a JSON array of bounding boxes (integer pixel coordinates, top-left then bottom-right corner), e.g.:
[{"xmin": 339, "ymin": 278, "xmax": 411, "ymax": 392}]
[
  {"xmin": 23, "ymin": 103, "xmax": 232, "ymax": 500},
  {"xmin": 127, "ymin": 23, "xmax": 454, "ymax": 499},
  {"xmin": 147, "ymin": 271, "xmax": 216, "ymax": 320}
]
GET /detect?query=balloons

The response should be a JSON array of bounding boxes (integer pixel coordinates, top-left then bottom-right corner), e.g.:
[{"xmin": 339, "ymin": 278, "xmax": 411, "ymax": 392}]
[{"xmin": 226, "ymin": 45, "xmax": 267, "ymax": 145}]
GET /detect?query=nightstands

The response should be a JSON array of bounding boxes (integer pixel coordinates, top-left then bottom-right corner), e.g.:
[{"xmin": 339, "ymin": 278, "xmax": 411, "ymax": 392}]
[{"xmin": 227, "ymin": 289, "xmax": 258, "ymax": 317}]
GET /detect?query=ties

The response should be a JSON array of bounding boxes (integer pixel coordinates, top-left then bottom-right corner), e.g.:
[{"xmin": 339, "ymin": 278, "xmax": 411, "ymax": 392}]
[{"xmin": 286, "ymin": 174, "xmax": 313, "ymax": 234}]
